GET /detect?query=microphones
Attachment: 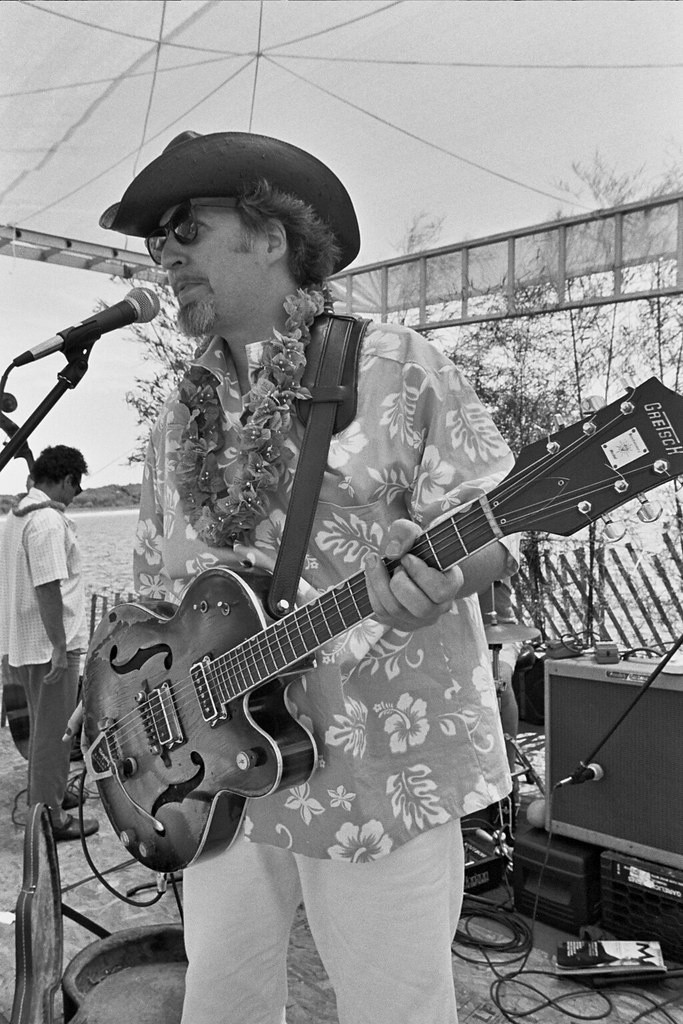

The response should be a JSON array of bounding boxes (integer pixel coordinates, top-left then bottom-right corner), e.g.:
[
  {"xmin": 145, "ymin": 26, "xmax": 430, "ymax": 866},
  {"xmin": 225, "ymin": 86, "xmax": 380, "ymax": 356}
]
[
  {"xmin": 555, "ymin": 764, "xmax": 604, "ymax": 787},
  {"xmin": 14, "ymin": 287, "xmax": 160, "ymax": 367}
]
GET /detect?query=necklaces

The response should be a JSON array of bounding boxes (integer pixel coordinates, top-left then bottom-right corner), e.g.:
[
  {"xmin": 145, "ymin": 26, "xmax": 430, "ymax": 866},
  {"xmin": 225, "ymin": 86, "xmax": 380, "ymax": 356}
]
[
  {"xmin": 173, "ymin": 284, "xmax": 336, "ymax": 548},
  {"xmin": 11, "ymin": 492, "xmax": 66, "ymax": 517}
]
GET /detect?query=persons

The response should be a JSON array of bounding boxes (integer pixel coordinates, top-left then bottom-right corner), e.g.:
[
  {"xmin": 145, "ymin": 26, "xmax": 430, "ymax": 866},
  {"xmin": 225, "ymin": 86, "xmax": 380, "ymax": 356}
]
[
  {"xmin": 0, "ymin": 444, "xmax": 99, "ymax": 841},
  {"xmin": 61, "ymin": 159, "xmax": 523, "ymax": 1024}
]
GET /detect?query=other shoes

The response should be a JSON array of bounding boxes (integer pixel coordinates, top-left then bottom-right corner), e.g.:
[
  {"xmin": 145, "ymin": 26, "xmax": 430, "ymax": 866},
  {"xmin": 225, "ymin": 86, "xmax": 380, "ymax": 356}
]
[
  {"xmin": 52, "ymin": 814, "xmax": 99, "ymax": 841},
  {"xmin": 62, "ymin": 791, "xmax": 86, "ymax": 810}
]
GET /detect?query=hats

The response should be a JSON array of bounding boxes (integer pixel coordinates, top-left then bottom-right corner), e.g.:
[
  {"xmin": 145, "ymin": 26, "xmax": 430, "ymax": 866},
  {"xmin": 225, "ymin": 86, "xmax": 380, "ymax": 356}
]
[{"xmin": 99, "ymin": 130, "xmax": 361, "ymax": 277}]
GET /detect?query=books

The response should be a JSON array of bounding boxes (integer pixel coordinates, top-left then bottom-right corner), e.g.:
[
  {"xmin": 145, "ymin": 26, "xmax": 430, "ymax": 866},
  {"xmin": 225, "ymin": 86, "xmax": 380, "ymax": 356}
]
[{"xmin": 555, "ymin": 940, "xmax": 668, "ymax": 977}]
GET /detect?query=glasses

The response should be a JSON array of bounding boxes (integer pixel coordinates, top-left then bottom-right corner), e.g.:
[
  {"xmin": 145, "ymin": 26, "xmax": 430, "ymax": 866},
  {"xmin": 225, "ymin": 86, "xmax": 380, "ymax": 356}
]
[
  {"xmin": 75, "ymin": 482, "xmax": 83, "ymax": 496},
  {"xmin": 145, "ymin": 200, "xmax": 239, "ymax": 265}
]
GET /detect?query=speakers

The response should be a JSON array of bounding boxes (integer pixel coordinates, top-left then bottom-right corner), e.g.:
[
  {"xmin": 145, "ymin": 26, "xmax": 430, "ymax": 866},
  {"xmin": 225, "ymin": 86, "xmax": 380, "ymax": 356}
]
[{"xmin": 544, "ymin": 653, "xmax": 683, "ymax": 870}]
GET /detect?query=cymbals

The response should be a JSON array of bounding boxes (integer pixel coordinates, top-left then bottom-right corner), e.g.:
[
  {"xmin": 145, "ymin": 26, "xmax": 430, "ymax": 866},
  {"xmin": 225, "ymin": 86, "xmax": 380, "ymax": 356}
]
[{"xmin": 483, "ymin": 622, "xmax": 544, "ymax": 646}]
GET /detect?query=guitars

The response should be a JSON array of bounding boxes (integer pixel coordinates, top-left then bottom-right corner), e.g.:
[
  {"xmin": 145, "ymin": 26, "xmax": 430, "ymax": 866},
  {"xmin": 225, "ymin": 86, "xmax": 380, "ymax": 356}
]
[{"xmin": 73, "ymin": 372, "xmax": 683, "ymax": 877}]
